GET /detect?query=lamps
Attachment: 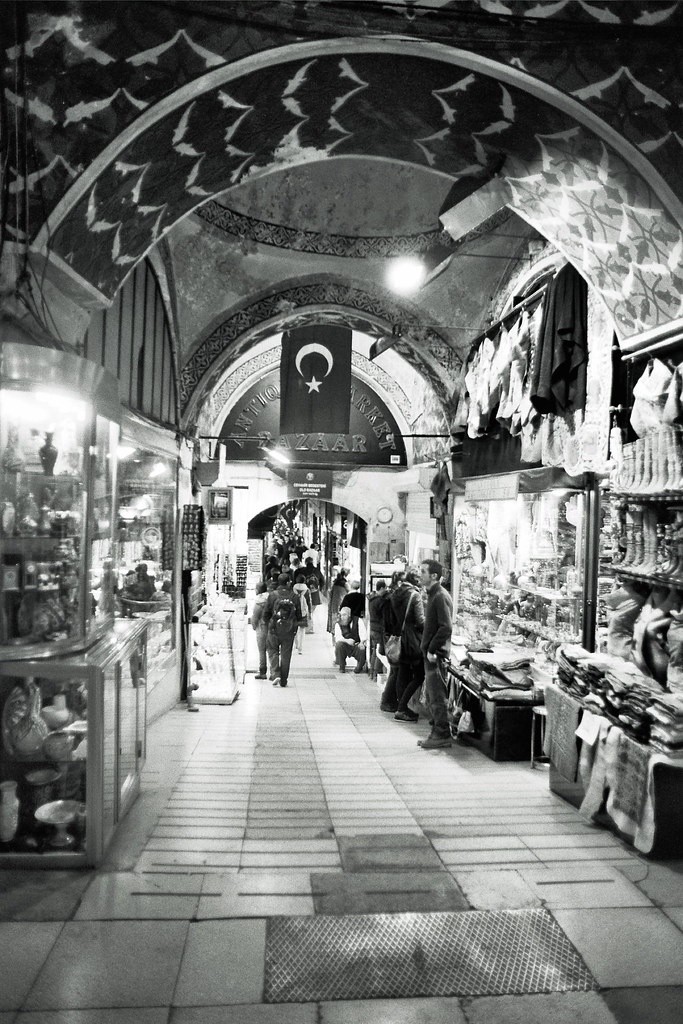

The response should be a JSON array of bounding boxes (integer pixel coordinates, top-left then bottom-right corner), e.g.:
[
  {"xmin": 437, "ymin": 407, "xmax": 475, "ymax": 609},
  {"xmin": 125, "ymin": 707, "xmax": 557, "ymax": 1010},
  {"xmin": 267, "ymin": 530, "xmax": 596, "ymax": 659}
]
[
  {"xmin": 198, "ymin": 434, "xmax": 293, "ymax": 466},
  {"xmin": 377, "ymin": 432, "xmax": 451, "ymax": 448},
  {"xmin": 368, "ymin": 323, "xmax": 485, "ymax": 360}
]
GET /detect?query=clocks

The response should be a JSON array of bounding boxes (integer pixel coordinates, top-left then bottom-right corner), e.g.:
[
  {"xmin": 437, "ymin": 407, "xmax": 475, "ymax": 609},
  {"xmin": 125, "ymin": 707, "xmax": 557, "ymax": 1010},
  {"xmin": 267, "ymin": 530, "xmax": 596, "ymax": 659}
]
[{"xmin": 377, "ymin": 507, "xmax": 393, "ymax": 523}]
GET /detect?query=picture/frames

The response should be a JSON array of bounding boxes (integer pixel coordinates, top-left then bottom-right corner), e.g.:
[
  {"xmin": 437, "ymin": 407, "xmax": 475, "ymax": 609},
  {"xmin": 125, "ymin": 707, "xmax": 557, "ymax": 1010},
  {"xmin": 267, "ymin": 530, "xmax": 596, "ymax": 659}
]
[{"xmin": 207, "ymin": 487, "xmax": 233, "ymax": 525}]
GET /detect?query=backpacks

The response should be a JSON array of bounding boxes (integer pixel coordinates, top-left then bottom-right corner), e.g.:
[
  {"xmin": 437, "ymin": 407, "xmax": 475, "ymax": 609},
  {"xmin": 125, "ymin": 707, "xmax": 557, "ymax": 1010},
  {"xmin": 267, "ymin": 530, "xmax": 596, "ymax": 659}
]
[{"xmin": 269, "ymin": 590, "xmax": 298, "ymax": 638}]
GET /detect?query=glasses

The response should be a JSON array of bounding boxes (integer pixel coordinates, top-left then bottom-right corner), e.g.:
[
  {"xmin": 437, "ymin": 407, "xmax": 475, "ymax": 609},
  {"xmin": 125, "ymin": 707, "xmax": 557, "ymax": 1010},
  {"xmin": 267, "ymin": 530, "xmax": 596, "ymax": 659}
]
[{"xmin": 288, "ymin": 581, "xmax": 292, "ymax": 584}]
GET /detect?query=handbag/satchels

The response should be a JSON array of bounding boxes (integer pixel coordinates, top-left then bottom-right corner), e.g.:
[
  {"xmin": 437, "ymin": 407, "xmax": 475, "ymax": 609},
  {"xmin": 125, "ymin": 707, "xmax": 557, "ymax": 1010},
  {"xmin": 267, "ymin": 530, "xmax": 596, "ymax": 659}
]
[
  {"xmin": 305, "ymin": 618, "xmax": 314, "ymax": 634},
  {"xmin": 384, "ymin": 635, "xmax": 402, "ymax": 663}
]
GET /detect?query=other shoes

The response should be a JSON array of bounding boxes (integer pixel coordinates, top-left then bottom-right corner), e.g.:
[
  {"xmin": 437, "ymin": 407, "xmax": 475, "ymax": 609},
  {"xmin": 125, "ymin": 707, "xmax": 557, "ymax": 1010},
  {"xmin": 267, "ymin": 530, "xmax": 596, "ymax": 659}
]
[
  {"xmin": 269, "ymin": 675, "xmax": 276, "ymax": 680},
  {"xmin": 279, "ymin": 681, "xmax": 286, "ymax": 686},
  {"xmin": 418, "ymin": 731, "xmax": 452, "ymax": 749},
  {"xmin": 255, "ymin": 674, "xmax": 267, "ymax": 679},
  {"xmin": 272, "ymin": 676, "xmax": 281, "ymax": 685},
  {"xmin": 381, "ymin": 704, "xmax": 398, "ymax": 711},
  {"xmin": 354, "ymin": 667, "xmax": 360, "ymax": 673},
  {"xmin": 339, "ymin": 664, "xmax": 345, "ymax": 672},
  {"xmin": 393, "ymin": 708, "xmax": 419, "ymax": 722}
]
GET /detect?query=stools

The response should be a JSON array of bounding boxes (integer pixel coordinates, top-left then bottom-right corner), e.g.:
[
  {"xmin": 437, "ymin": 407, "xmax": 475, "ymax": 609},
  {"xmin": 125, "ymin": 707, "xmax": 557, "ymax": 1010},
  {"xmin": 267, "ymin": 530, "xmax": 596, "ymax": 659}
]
[{"xmin": 531, "ymin": 706, "xmax": 550, "ymax": 772}]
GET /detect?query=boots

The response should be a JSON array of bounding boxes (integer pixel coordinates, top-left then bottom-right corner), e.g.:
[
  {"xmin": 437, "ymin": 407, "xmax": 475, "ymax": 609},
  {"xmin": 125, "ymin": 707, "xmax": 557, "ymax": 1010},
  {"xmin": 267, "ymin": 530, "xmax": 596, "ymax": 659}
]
[{"xmin": 612, "ymin": 430, "xmax": 683, "ymax": 586}]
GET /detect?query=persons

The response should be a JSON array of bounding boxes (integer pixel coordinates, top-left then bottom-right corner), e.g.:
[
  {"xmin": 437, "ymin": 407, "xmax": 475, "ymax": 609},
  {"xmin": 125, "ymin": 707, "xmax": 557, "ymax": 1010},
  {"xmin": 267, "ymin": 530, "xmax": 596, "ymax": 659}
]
[
  {"xmin": 365, "ymin": 569, "xmax": 426, "ymax": 722},
  {"xmin": 248, "ymin": 521, "xmax": 324, "ymax": 687},
  {"xmin": 327, "ymin": 557, "xmax": 366, "ymax": 646},
  {"xmin": 115, "ymin": 564, "xmax": 152, "ymax": 617},
  {"xmin": 335, "ymin": 607, "xmax": 368, "ymax": 673},
  {"xmin": 417, "ymin": 560, "xmax": 452, "ymax": 750}
]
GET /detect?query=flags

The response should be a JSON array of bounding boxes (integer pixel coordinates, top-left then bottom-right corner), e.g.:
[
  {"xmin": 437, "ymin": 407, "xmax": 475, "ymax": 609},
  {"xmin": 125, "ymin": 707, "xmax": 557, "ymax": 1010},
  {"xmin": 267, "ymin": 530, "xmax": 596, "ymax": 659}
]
[{"xmin": 280, "ymin": 325, "xmax": 352, "ymax": 435}]
